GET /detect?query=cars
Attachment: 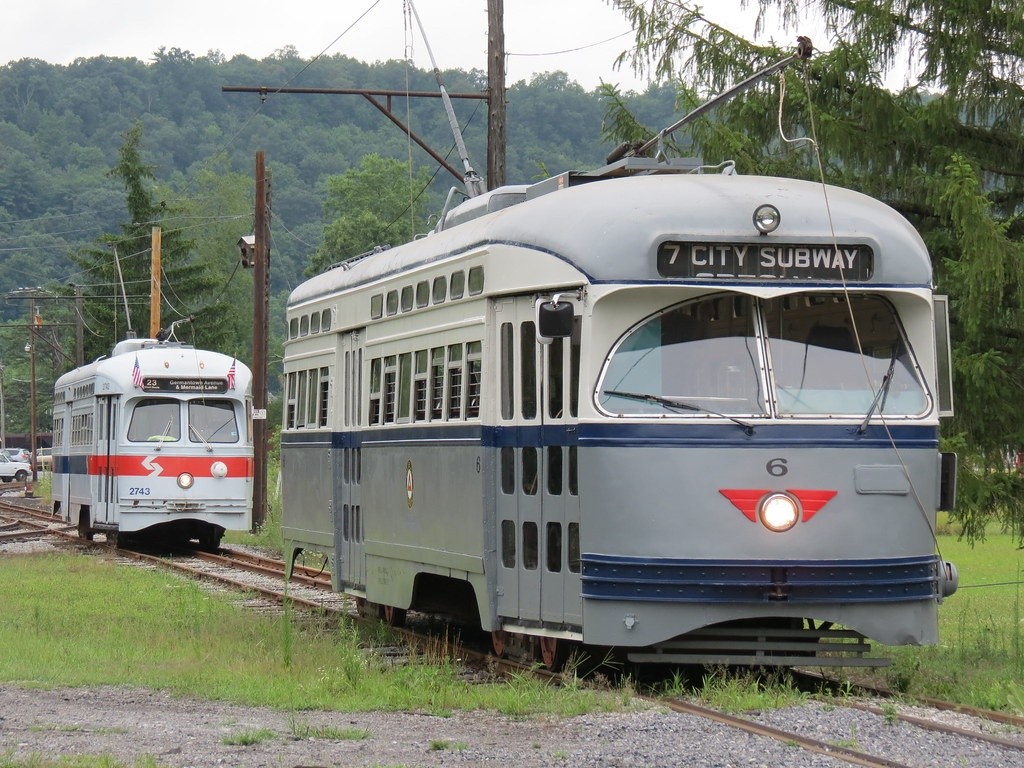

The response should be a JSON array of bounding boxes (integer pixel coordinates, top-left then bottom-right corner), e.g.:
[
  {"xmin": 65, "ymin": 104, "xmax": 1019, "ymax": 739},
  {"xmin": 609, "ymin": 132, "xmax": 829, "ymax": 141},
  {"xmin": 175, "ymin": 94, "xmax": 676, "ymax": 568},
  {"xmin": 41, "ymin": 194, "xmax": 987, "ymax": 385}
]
[
  {"xmin": 35, "ymin": 447, "xmax": 53, "ymax": 471},
  {"xmin": 0, "ymin": 447, "xmax": 32, "ymax": 483}
]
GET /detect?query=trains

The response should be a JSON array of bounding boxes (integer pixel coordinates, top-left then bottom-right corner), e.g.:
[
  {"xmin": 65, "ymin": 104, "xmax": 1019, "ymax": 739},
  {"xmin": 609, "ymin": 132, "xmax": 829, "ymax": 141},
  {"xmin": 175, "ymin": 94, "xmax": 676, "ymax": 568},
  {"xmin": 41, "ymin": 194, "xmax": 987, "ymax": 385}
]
[
  {"xmin": 278, "ymin": 1, "xmax": 960, "ymax": 674},
  {"xmin": 50, "ymin": 247, "xmax": 253, "ymax": 555}
]
